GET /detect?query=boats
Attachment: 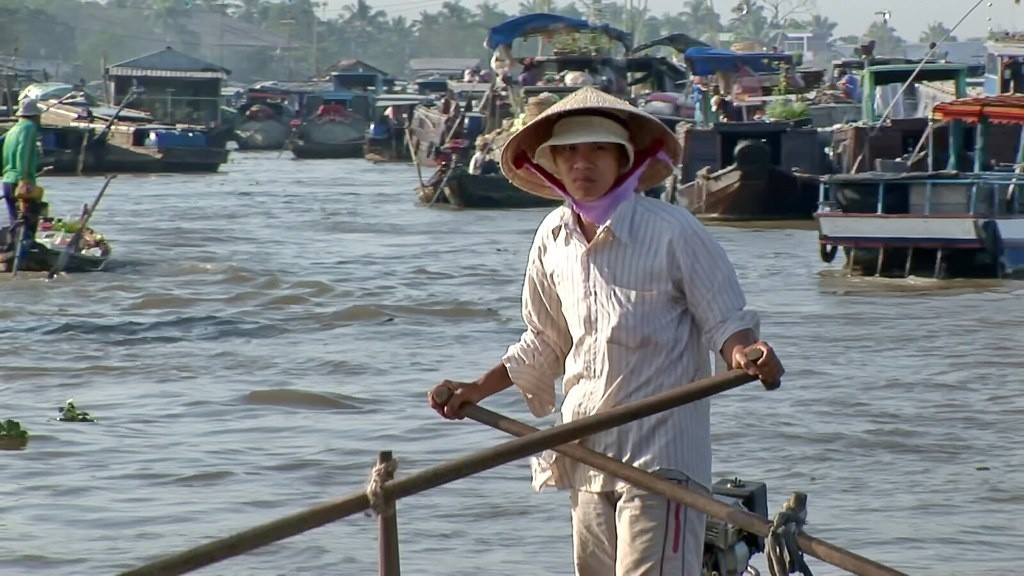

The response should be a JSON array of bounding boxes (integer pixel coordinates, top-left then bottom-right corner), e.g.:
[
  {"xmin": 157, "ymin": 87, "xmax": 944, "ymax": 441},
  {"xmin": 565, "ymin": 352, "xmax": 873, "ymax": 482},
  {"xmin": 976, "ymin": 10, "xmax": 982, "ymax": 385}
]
[
  {"xmin": 0, "ymin": 0, "xmax": 1024, "ymax": 282},
  {"xmin": 0, "ymin": 215, "xmax": 113, "ymax": 275}
]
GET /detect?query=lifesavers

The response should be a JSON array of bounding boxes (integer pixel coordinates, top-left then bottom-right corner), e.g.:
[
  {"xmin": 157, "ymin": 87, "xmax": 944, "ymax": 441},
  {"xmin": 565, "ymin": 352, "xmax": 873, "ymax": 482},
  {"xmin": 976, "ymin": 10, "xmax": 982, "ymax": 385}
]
[
  {"xmin": 821, "ymin": 244, "xmax": 837, "ymax": 262},
  {"xmin": 734, "ymin": 142, "xmax": 771, "ymax": 164},
  {"xmin": 983, "ymin": 221, "xmax": 1003, "ymax": 257}
]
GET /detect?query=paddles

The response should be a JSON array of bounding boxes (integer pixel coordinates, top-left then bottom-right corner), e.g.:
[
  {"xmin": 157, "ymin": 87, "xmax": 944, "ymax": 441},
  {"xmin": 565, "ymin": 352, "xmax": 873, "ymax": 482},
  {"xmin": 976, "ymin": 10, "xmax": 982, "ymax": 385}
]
[
  {"xmin": 48, "ymin": 174, "xmax": 118, "ymax": 279},
  {"xmin": 434, "ymin": 387, "xmax": 909, "ymax": 576},
  {"xmin": 0, "ymin": 166, "xmax": 54, "ymax": 199},
  {"xmin": 114, "ymin": 349, "xmax": 781, "ymax": 576}
]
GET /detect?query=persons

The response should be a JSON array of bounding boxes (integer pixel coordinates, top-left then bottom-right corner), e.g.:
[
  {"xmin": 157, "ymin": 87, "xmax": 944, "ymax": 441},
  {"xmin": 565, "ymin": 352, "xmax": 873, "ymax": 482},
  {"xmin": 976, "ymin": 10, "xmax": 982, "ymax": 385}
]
[
  {"xmin": 463, "ymin": 57, "xmax": 540, "ymax": 105},
  {"xmin": 688, "ymin": 75, "xmax": 744, "ymax": 125},
  {"xmin": 771, "ymin": 45, "xmax": 778, "ymax": 54},
  {"xmin": 425, "ymin": 85, "xmax": 786, "ymax": 576},
  {"xmin": 835, "ymin": 70, "xmax": 858, "ymax": 99},
  {"xmin": 1004, "ymin": 57, "xmax": 1021, "ymax": 93},
  {"xmin": 2, "ymin": 98, "xmax": 46, "ymax": 255}
]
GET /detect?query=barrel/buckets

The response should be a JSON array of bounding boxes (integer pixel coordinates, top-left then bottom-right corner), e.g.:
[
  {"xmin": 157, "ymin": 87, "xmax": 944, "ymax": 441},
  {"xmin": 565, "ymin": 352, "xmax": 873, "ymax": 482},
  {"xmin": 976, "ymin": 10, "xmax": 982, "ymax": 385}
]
[
  {"xmin": 369, "ymin": 122, "xmax": 385, "ymax": 136},
  {"xmin": 461, "ymin": 116, "xmax": 486, "ymax": 134}
]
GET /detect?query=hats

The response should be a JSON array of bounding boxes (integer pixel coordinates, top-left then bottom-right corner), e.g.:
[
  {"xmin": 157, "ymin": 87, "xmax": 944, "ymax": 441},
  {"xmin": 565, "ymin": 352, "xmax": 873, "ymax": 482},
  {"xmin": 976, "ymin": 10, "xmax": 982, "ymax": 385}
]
[
  {"xmin": 15, "ymin": 97, "xmax": 49, "ymax": 116},
  {"xmin": 499, "ymin": 84, "xmax": 682, "ymax": 201},
  {"xmin": 710, "ymin": 95, "xmax": 721, "ymax": 112}
]
